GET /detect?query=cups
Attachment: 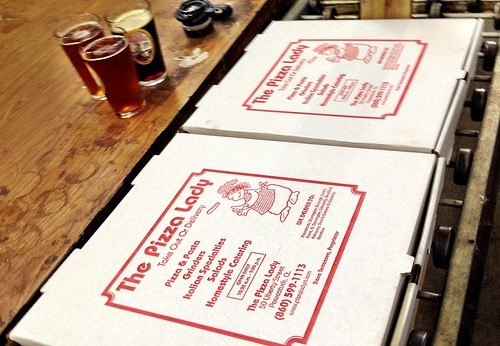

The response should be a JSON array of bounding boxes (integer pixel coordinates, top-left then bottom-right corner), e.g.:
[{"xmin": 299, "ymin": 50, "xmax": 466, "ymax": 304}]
[
  {"xmin": 105, "ymin": 0, "xmax": 168, "ymax": 88},
  {"xmin": 53, "ymin": 13, "xmax": 116, "ymax": 100},
  {"xmin": 78, "ymin": 24, "xmax": 147, "ymax": 120}
]
